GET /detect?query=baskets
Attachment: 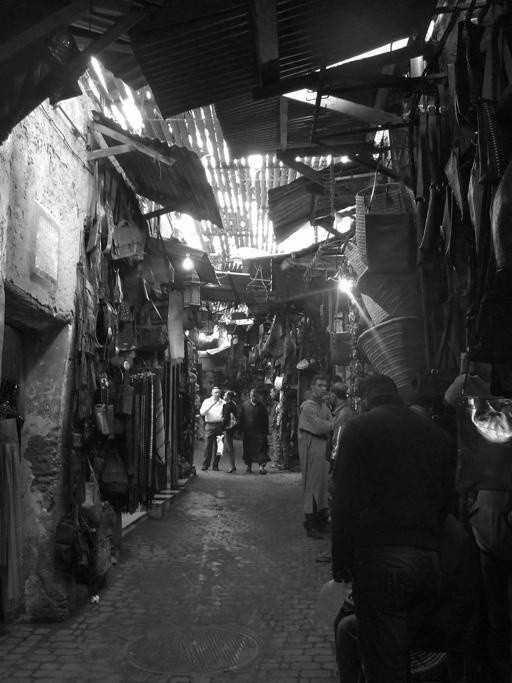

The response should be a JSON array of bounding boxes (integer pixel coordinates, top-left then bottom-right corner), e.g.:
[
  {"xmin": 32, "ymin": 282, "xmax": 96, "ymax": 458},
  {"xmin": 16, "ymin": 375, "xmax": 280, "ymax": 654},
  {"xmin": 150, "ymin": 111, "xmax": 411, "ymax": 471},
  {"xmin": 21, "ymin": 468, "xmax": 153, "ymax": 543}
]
[
  {"xmin": 354, "ymin": 182, "xmax": 416, "ymax": 272},
  {"xmin": 353, "ymin": 265, "xmax": 422, "ymax": 324},
  {"xmin": 354, "ymin": 315, "xmax": 425, "ymax": 392}
]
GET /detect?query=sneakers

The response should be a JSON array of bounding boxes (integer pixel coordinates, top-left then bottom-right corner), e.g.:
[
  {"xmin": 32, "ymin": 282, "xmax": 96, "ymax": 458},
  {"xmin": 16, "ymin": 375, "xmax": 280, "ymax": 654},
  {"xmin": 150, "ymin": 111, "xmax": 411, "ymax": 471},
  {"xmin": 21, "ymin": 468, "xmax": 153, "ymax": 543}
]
[{"xmin": 201, "ymin": 465, "xmax": 268, "ymax": 475}]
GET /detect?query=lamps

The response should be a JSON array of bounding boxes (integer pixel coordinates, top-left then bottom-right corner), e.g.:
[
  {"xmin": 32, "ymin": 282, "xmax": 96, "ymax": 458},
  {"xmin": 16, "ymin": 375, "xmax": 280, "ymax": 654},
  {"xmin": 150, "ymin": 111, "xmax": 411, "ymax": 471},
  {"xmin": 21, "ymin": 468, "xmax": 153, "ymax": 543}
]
[
  {"xmin": 333, "ymin": 212, "xmax": 353, "ymax": 235},
  {"xmin": 337, "ymin": 268, "xmax": 357, "ymax": 293},
  {"xmin": 182, "ymin": 253, "xmax": 194, "ymax": 270}
]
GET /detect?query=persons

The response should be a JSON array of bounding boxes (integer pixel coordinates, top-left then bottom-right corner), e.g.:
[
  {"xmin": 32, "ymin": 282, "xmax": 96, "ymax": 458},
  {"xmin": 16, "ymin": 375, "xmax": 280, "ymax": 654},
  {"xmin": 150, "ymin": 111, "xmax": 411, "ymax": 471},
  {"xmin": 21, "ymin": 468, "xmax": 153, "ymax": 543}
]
[
  {"xmin": 219, "ymin": 390, "xmax": 239, "ymax": 473},
  {"xmin": 199, "ymin": 386, "xmax": 226, "ymax": 470},
  {"xmin": 298, "ymin": 373, "xmax": 511, "ymax": 681},
  {"xmin": 239, "ymin": 387, "xmax": 271, "ymax": 474}
]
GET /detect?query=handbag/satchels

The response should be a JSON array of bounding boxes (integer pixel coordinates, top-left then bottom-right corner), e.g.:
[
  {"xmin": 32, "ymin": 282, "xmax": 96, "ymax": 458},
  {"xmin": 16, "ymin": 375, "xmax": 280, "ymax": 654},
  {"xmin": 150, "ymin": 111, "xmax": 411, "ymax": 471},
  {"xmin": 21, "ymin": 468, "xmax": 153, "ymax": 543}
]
[
  {"xmin": 364, "ymin": 146, "xmax": 415, "ymax": 281},
  {"xmin": 417, "ymin": 73, "xmax": 511, "ymax": 493},
  {"xmin": 220, "ymin": 406, "xmax": 237, "ymax": 430},
  {"xmin": 74, "ymin": 204, "xmax": 170, "ymax": 508},
  {"xmin": 216, "ymin": 436, "xmax": 224, "ymax": 456}
]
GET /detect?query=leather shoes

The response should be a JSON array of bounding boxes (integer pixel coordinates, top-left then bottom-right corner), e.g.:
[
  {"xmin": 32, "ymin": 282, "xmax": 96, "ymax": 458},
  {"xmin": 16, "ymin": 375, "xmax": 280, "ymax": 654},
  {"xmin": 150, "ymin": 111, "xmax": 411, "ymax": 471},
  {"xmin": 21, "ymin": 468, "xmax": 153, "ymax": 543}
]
[
  {"xmin": 317, "ymin": 521, "xmax": 331, "ymax": 533},
  {"xmin": 306, "ymin": 527, "xmax": 322, "ymax": 540}
]
